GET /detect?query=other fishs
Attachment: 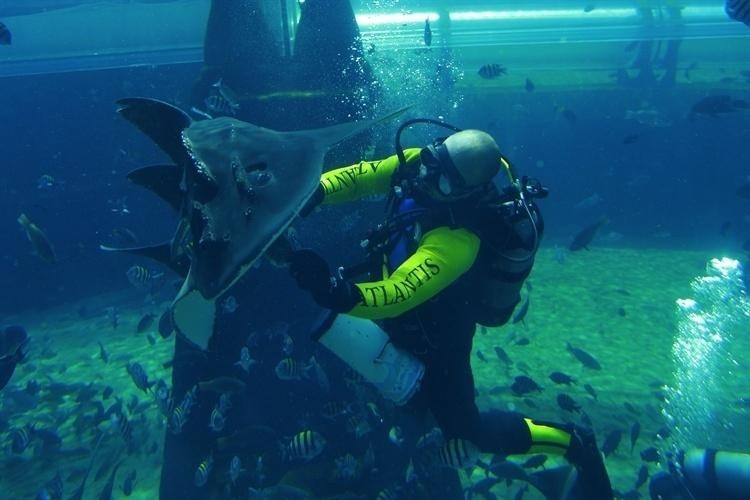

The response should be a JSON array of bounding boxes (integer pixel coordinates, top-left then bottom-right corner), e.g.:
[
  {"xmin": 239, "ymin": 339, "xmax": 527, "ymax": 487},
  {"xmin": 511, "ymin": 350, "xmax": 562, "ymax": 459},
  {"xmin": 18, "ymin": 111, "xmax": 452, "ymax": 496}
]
[{"xmin": 0, "ymin": 95, "xmax": 686, "ymax": 500}]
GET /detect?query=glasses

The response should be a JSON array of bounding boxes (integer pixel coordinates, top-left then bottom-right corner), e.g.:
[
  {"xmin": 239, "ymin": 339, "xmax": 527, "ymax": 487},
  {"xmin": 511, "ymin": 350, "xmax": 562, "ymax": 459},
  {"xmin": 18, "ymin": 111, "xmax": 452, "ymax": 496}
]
[{"xmin": 421, "ymin": 138, "xmax": 465, "ymax": 198}]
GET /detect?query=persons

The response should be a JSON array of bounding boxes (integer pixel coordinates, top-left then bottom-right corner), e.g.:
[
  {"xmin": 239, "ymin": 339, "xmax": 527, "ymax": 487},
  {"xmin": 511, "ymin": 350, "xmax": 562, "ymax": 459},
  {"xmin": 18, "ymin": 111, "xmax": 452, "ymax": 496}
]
[{"xmin": 292, "ymin": 128, "xmax": 613, "ymax": 500}]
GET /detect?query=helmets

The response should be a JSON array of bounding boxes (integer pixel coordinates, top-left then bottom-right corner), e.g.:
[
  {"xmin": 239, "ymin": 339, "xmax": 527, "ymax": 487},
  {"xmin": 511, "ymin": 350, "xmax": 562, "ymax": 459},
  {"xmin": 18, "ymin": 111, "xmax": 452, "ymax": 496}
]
[{"xmin": 441, "ymin": 129, "xmax": 500, "ymax": 194}]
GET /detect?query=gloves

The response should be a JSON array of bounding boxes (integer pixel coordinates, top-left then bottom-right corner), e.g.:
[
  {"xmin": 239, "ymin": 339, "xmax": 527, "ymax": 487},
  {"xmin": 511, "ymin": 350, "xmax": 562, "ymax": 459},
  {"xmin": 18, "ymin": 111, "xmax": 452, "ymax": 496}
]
[{"xmin": 295, "ymin": 251, "xmax": 356, "ymax": 313}]
